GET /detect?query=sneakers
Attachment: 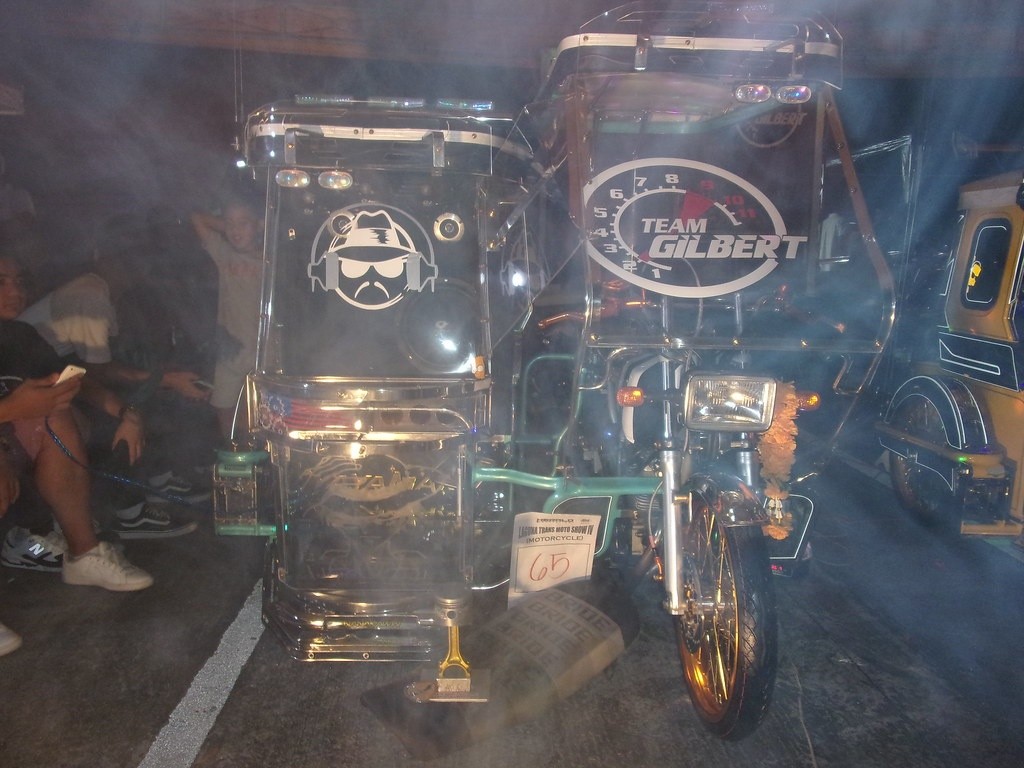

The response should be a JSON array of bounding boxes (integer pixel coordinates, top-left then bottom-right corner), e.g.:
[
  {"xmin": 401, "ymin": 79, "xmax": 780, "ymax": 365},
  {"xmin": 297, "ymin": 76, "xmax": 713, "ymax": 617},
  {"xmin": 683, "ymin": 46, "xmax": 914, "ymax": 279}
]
[
  {"xmin": 146, "ymin": 470, "xmax": 212, "ymax": 505},
  {"xmin": 0, "ymin": 520, "xmax": 68, "ymax": 573},
  {"xmin": 39, "ymin": 514, "xmax": 126, "ymax": 553},
  {"xmin": 108, "ymin": 502, "xmax": 199, "ymax": 540},
  {"xmin": 61, "ymin": 540, "xmax": 156, "ymax": 591},
  {"xmin": 0, "ymin": 623, "xmax": 23, "ymax": 656}
]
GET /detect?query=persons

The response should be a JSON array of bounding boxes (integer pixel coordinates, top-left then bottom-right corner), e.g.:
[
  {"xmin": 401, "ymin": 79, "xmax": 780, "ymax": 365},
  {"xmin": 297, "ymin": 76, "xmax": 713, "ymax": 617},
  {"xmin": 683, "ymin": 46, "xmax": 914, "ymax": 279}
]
[
  {"xmin": 0, "ymin": 217, "xmax": 211, "ymax": 656},
  {"xmin": 190, "ymin": 203, "xmax": 269, "ymax": 448}
]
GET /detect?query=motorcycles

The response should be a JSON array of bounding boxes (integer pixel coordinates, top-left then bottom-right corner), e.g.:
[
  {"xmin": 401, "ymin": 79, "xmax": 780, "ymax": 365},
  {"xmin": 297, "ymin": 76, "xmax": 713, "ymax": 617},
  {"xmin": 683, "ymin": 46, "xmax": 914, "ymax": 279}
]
[{"xmin": 211, "ymin": 0, "xmax": 1023, "ymax": 744}]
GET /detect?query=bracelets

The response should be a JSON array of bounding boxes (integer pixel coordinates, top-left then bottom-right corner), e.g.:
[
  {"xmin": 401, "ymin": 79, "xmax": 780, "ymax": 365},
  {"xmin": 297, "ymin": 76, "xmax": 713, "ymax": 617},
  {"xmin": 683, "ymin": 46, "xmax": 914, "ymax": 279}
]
[
  {"xmin": 119, "ymin": 403, "xmax": 135, "ymax": 415},
  {"xmin": 151, "ymin": 371, "xmax": 163, "ymax": 383}
]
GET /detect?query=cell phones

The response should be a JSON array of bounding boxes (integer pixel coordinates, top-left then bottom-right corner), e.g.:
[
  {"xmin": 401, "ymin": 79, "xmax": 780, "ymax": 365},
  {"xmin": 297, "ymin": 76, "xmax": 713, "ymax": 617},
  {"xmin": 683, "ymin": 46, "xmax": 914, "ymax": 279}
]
[
  {"xmin": 192, "ymin": 379, "xmax": 215, "ymax": 393},
  {"xmin": 53, "ymin": 365, "xmax": 86, "ymax": 388}
]
